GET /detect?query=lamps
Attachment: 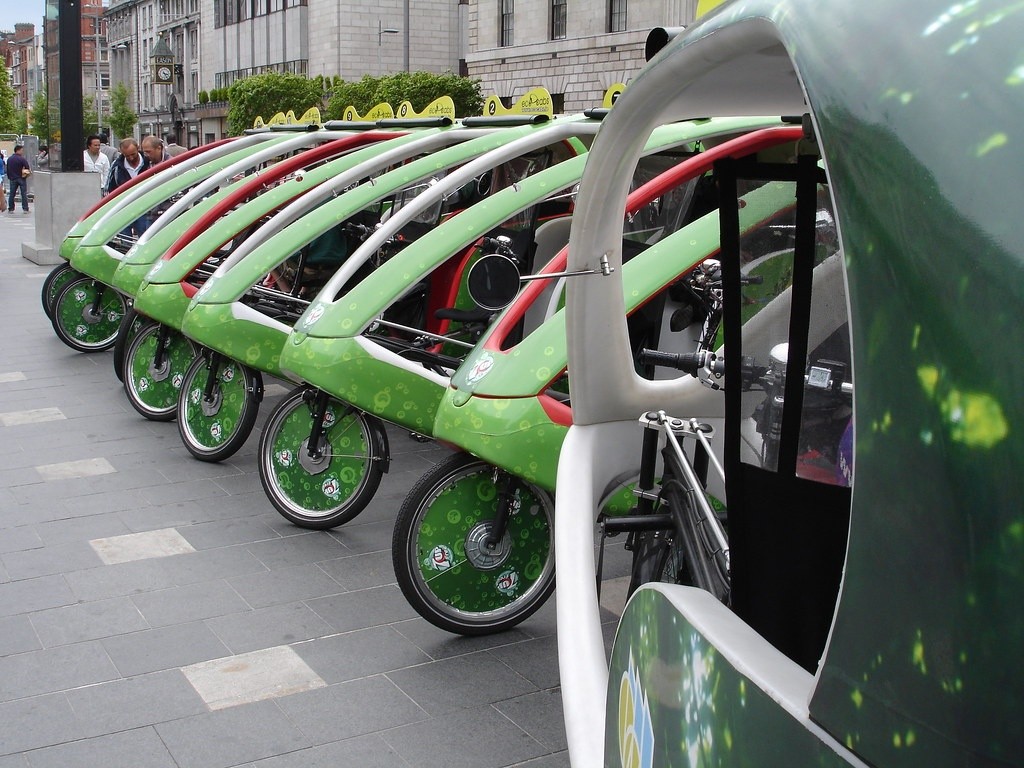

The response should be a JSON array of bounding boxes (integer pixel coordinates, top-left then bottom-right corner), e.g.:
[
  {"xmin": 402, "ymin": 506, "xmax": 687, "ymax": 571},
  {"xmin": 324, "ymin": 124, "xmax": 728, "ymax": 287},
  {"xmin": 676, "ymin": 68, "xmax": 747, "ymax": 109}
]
[{"xmin": 378, "ymin": 30, "xmax": 399, "ymax": 34}]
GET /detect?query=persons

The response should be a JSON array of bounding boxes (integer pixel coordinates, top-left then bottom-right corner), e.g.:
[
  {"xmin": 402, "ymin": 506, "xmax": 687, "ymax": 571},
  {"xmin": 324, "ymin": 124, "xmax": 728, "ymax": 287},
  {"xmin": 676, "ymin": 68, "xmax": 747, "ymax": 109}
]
[
  {"xmin": 0, "ymin": 145, "xmax": 50, "ymax": 218},
  {"xmin": 84, "ymin": 134, "xmax": 220, "ymax": 247}
]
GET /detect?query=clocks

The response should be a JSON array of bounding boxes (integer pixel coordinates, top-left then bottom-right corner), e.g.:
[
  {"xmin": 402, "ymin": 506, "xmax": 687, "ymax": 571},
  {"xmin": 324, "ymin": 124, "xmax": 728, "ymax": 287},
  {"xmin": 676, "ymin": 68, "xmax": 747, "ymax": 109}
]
[{"xmin": 156, "ymin": 65, "xmax": 174, "ymax": 82}]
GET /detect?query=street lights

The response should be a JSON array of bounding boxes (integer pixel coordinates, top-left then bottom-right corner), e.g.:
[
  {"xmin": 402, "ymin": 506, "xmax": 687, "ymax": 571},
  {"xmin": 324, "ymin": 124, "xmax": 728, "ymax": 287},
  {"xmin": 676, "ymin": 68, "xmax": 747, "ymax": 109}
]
[
  {"xmin": 7, "ymin": 40, "xmax": 39, "ymax": 95},
  {"xmin": 83, "ymin": 4, "xmax": 110, "ymax": 135}
]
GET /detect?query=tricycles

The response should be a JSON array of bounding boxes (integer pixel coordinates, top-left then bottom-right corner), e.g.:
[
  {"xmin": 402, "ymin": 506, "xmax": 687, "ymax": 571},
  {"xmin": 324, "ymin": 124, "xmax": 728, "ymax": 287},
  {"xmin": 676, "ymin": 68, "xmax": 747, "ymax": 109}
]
[{"xmin": 36, "ymin": 1, "xmax": 1024, "ymax": 768}]
